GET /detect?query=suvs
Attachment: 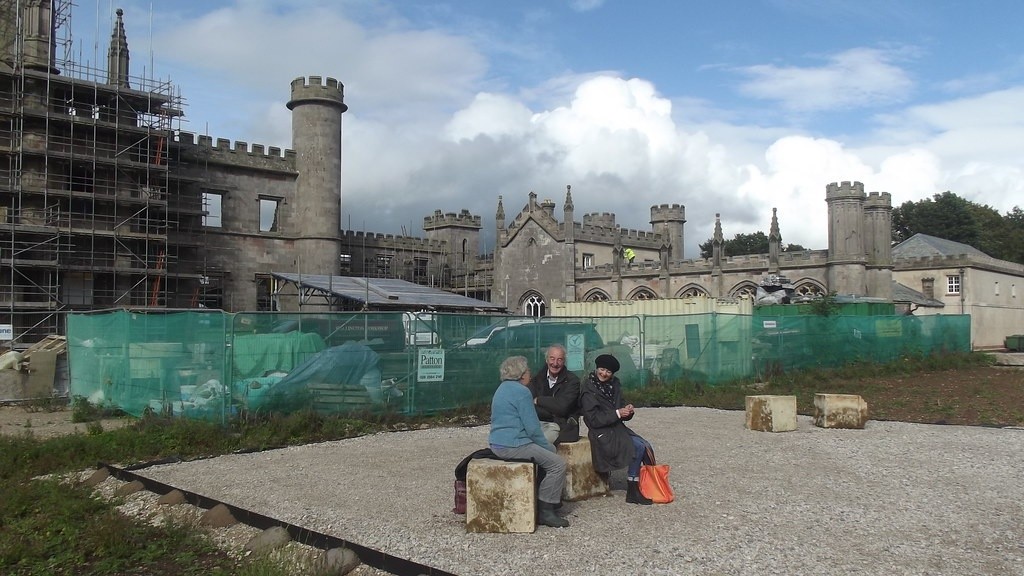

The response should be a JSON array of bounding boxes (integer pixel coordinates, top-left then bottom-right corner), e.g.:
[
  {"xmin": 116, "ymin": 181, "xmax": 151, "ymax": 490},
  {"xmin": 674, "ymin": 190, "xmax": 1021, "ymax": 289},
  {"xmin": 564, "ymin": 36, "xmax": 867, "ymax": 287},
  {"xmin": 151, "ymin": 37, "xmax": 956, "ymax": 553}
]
[{"xmin": 444, "ymin": 321, "xmax": 605, "ymax": 365}]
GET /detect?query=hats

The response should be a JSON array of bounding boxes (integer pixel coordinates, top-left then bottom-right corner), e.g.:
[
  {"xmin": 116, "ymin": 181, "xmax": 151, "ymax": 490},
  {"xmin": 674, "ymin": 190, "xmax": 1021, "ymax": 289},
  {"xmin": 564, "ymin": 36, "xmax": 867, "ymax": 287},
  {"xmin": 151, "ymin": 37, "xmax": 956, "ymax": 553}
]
[{"xmin": 595, "ymin": 353, "xmax": 620, "ymax": 374}]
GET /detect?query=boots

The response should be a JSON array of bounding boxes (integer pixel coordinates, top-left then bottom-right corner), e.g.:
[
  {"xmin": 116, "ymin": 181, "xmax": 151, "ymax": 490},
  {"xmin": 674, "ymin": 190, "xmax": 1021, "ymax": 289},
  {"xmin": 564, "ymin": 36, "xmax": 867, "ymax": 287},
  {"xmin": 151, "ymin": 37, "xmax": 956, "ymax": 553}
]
[
  {"xmin": 537, "ymin": 501, "xmax": 569, "ymax": 527},
  {"xmin": 626, "ymin": 479, "xmax": 653, "ymax": 505}
]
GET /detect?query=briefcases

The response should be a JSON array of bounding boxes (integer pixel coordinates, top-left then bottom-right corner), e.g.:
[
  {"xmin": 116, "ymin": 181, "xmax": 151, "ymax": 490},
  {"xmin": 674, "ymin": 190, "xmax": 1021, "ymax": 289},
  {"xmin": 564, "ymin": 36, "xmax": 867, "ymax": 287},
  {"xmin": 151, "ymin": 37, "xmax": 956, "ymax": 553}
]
[{"xmin": 454, "ymin": 481, "xmax": 466, "ymax": 514}]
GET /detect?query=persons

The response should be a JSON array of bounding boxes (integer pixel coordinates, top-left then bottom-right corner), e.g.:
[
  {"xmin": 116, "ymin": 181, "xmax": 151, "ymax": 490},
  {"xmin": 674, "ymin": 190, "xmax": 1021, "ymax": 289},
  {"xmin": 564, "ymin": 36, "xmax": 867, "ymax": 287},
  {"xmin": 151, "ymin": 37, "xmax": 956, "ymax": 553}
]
[
  {"xmin": 577, "ymin": 354, "xmax": 657, "ymax": 504},
  {"xmin": 526, "ymin": 343, "xmax": 581, "ymax": 449},
  {"xmin": 623, "ymin": 246, "xmax": 636, "ymax": 263},
  {"xmin": 489, "ymin": 356, "xmax": 571, "ymax": 528},
  {"xmin": 903, "ymin": 305, "xmax": 913, "ymax": 315}
]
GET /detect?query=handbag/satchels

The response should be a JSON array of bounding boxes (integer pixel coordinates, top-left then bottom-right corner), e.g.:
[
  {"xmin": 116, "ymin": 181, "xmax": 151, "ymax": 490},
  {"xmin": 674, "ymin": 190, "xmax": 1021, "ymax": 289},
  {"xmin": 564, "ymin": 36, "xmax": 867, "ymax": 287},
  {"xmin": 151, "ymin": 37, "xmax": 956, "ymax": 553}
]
[{"xmin": 639, "ymin": 446, "xmax": 674, "ymax": 503}]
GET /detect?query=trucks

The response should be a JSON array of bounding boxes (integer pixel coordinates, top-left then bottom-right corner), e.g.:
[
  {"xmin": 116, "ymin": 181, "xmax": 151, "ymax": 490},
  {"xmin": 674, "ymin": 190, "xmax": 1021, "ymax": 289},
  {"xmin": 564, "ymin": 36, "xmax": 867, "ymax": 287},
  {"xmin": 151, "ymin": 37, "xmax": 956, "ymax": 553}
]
[{"xmin": 403, "ymin": 311, "xmax": 439, "ymax": 348}]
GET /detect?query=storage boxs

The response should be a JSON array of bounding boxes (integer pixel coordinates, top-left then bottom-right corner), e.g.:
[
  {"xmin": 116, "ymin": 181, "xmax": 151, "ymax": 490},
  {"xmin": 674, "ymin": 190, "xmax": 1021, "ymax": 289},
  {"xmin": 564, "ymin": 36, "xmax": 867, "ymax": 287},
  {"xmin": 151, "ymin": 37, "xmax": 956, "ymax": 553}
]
[{"xmin": 550, "ymin": 298, "xmax": 899, "ymax": 384}]
